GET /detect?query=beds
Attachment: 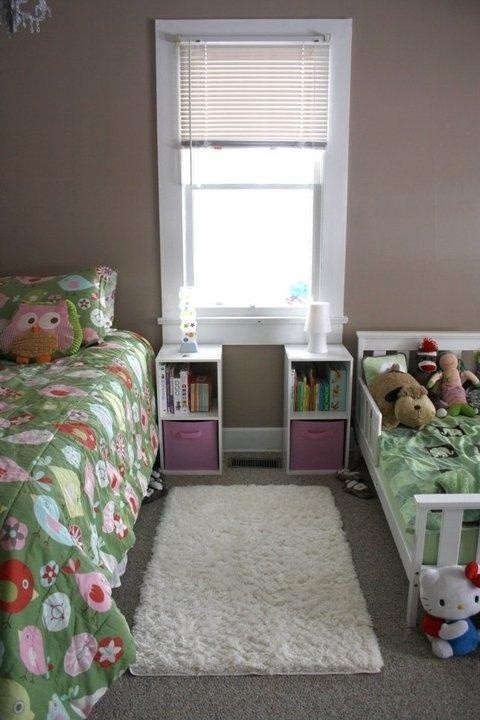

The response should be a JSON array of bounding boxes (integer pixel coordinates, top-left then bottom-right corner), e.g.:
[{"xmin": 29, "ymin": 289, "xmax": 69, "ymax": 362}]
[
  {"xmin": 0, "ymin": 327, "xmax": 155, "ymax": 719},
  {"xmin": 353, "ymin": 328, "xmax": 480, "ymax": 627}
]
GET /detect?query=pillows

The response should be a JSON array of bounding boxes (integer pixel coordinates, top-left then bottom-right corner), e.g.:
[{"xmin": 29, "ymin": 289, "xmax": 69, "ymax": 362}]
[{"xmin": 0, "ymin": 262, "xmax": 120, "ymax": 346}]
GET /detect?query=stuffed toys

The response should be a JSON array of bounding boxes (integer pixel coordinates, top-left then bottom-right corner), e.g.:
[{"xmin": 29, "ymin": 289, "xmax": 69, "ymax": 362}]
[
  {"xmin": 427, "ymin": 350, "xmax": 480, "ymax": 417},
  {"xmin": 409, "ymin": 337, "xmax": 448, "ymax": 418},
  {"xmin": 0, "ymin": 299, "xmax": 83, "ymax": 364},
  {"xmin": 419, "ymin": 561, "xmax": 480, "ymax": 658},
  {"xmin": 373, "ymin": 364, "xmax": 437, "ymax": 430}
]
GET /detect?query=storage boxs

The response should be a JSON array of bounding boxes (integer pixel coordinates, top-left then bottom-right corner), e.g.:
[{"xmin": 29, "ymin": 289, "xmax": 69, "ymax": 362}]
[
  {"xmin": 290, "ymin": 421, "xmax": 345, "ymax": 471},
  {"xmin": 162, "ymin": 422, "xmax": 219, "ymax": 473}
]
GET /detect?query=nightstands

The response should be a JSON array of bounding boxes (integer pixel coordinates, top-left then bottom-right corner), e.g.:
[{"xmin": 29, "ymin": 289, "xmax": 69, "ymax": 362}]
[
  {"xmin": 282, "ymin": 343, "xmax": 354, "ymax": 475},
  {"xmin": 154, "ymin": 342, "xmax": 225, "ymax": 476}
]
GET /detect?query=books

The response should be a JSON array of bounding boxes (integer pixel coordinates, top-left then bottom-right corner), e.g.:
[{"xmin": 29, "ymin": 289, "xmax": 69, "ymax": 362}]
[
  {"xmin": 292, "ymin": 366, "xmax": 345, "ymax": 412},
  {"xmin": 163, "ymin": 366, "xmax": 211, "ymax": 412}
]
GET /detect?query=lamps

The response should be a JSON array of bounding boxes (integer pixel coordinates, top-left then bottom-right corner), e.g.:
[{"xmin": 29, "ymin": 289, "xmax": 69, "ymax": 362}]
[
  {"xmin": 303, "ymin": 301, "xmax": 333, "ymax": 354},
  {"xmin": 176, "ymin": 286, "xmax": 200, "ymax": 353}
]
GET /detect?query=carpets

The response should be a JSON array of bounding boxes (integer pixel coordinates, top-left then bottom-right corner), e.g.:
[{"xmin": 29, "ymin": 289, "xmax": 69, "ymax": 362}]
[{"xmin": 125, "ymin": 484, "xmax": 383, "ymax": 678}]
